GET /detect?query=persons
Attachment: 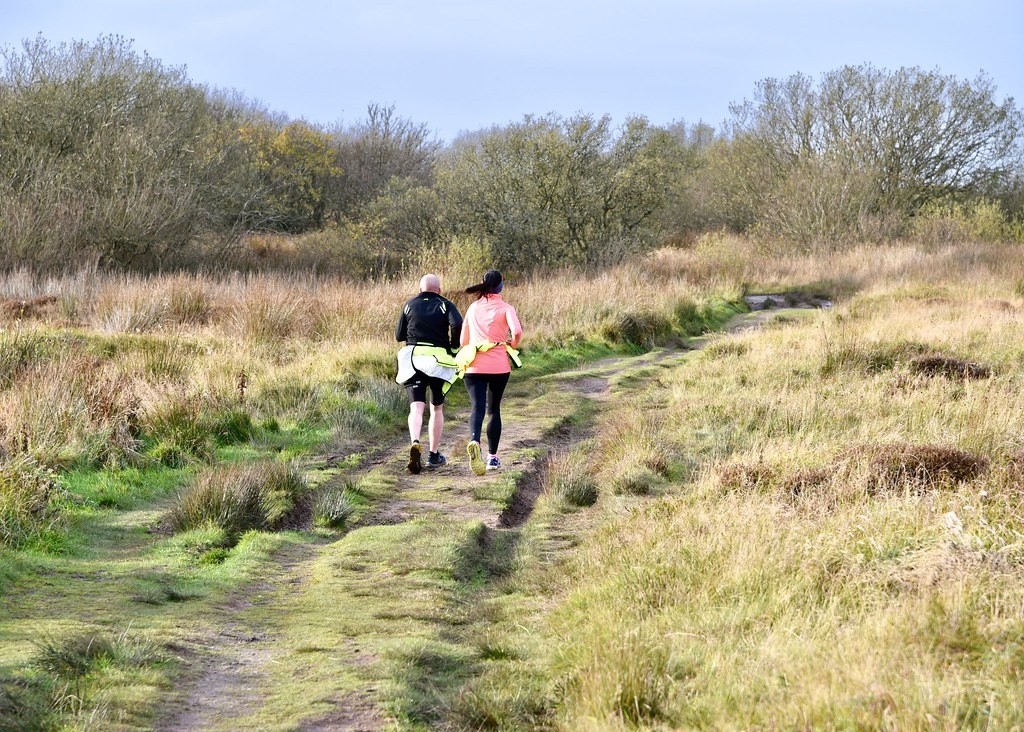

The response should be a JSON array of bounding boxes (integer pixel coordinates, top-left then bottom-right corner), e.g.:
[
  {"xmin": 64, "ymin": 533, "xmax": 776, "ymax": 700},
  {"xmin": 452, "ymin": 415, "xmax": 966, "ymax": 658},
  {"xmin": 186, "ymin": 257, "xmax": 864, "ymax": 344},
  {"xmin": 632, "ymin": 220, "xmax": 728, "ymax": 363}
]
[
  {"xmin": 395, "ymin": 273, "xmax": 464, "ymax": 474},
  {"xmin": 456, "ymin": 270, "xmax": 523, "ymax": 477}
]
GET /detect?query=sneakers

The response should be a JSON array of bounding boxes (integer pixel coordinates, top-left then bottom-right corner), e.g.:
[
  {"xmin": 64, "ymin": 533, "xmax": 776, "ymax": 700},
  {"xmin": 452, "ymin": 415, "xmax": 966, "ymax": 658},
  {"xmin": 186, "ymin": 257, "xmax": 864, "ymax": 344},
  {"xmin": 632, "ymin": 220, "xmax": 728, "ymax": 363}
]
[
  {"xmin": 467, "ymin": 440, "xmax": 486, "ymax": 476},
  {"xmin": 407, "ymin": 441, "xmax": 422, "ymax": 474},
  {"xmin": 488, "ymin": 456, "xmax": 501, "ymax": 470},
  {"xmin": 426, "ymin": 453, "xmax": 446, "ymax": 468}
]
